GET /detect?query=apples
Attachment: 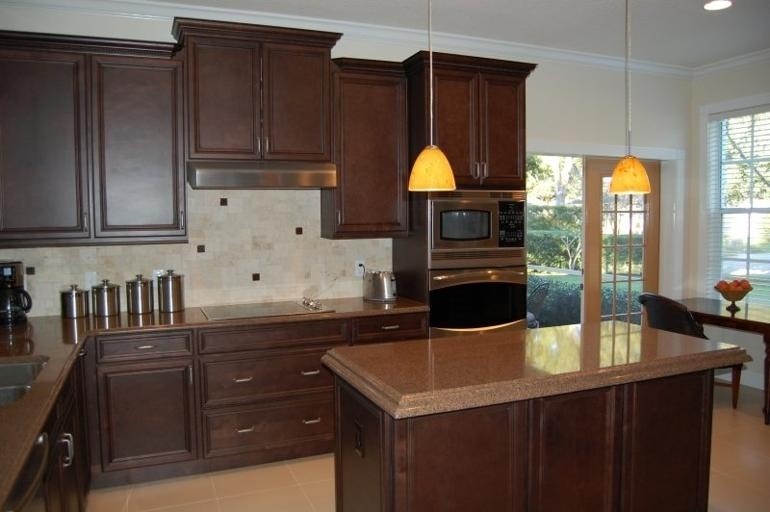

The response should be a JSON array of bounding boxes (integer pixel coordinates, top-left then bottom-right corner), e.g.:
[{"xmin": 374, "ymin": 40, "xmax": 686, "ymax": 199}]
[{"xmin": 717, "ymin": 280, "xmax": 750, "ymax": 290}]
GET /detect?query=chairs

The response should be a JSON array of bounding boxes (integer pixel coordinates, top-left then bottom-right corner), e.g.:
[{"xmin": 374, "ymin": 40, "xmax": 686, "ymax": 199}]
[{"xmin": 637, "ymin": 292, "xmax": 745, "ymax": 409}]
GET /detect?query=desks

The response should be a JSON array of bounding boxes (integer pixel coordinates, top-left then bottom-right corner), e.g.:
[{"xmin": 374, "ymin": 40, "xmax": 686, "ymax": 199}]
[{"xmin": 674, "ymin": 297, "xmax": 770, "ymax": 425}]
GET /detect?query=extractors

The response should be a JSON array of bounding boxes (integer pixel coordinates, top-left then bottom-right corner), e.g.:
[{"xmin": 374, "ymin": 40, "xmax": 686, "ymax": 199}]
[{"xmin": 182, "ymin": 159, "xmax": 340, "ymax": 188}]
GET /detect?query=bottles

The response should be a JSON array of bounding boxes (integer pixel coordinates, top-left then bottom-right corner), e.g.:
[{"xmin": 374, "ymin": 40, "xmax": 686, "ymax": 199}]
[
  {"xmin": 156, "ymin": 269, "xmax": 187, "ymax": 314},
  {"xmin": 90, "ymin": 279, "xmax": 122, "ymax": 317},
  {"xmin": 58, "ymin": 283, "xmax": 90, "ymax": 319},
  {"xmin": 124, "ymin": 274, "xmax": 155, "ymax": 315}
]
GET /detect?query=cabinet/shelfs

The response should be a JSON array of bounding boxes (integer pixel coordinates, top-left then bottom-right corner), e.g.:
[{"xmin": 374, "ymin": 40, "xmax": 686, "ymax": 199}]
[
  {"xmin": 95, "ymin": 330, "xmax": 198, "ymax": 473},
  {"xmin": 0, "ymin": 44, "xmax": 191, "ymax": 250},
  {"xmin": 350, "ymin": 312, "xmax": 428, "ymax": 343},
  {"xmin": 409, "ymin": 58, "xmax": 526, "ymax": 189},
  {"xmin": 198, "ymin": 318, "xmax": 350, "ymax": 460},
  {"xmin": 185, "ymin": 34, "xmax": 330, "ymax": 162},
  {"xmin": 1, "ymin": 341, "xmax": 87, "ymax": 511},
  {"xmin": 320, "ymin": 69, "xmax": 412, "ymax": 239}
]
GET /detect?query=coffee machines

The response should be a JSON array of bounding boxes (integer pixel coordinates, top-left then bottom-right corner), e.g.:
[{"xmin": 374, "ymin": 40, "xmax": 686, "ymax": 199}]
[{"xmin": 0, "ymin": 258, "xmax": 32, "ymax": 326}]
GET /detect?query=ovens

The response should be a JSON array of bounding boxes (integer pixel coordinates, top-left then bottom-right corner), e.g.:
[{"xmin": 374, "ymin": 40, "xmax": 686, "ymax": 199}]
[{"xmin": 389, "ymin": 187, "xmax": 529, "ymax": 341}]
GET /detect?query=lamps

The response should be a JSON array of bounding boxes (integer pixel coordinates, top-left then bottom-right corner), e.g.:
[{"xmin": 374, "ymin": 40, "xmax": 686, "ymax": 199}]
[
  {"xmin": 608, "ymin": 1, "xmax": 651, "ymax": 195},
  {"xmin": 406, "ymin": 1, "xmax": 456, "ymax": 193}
]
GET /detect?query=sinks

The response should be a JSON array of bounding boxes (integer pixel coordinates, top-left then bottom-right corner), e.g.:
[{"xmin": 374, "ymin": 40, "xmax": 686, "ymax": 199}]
[
  {"xmin": 0, "ymin": 355, "xmax": 51, "ymax": 385},
  {"xmin": 0, "ymin": 384, "xmax": 30, "ymax": 408}
]
